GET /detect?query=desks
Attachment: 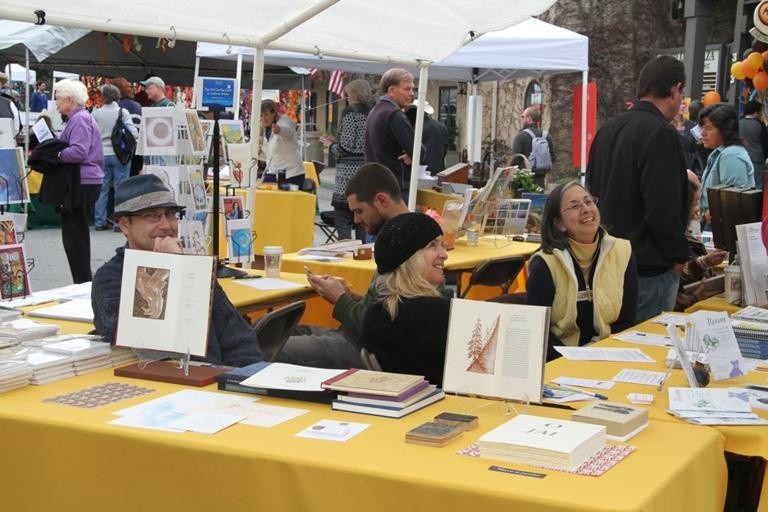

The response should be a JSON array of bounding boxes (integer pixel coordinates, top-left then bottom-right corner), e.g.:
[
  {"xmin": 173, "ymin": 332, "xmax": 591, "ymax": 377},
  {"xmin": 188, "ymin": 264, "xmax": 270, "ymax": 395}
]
[{"xmin": 1, "ymin": 160, "xmax": 767, "ymax": 511}]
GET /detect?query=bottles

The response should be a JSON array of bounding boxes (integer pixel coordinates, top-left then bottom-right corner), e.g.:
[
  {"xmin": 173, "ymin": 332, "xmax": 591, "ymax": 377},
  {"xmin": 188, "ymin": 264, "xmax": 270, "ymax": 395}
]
[
  {"xmin": 278, "ymin": 168, "xmax": 287, "ymax": 189},
  {"xmin": 466, "ymin": 221, "xmax": 479, "ymax": 244}
]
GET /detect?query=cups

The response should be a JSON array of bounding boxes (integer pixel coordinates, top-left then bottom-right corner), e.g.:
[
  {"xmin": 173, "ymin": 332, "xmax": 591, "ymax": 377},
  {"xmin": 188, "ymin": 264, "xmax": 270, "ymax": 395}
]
[
  {"xmin": 262, "ymin": 247, "xmax": 283, "ymax": 277},
  {"xmin": 444, "ymin": 222, "xmax": 458, "ymax": 250},
  {"xmin": 723, "ymin": 267, "xmax": 744, "ymax": 306}
]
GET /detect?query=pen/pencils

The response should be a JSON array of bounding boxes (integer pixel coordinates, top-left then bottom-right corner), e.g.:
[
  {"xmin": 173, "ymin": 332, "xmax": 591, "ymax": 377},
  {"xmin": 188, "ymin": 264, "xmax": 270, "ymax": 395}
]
[
  {"xmin": 0, "ymin": 306, "xmax": 24, "ymax": 315},
  {"xmin": 559, "ymin": 384, "xmax": 609, "ymax": 400},
  {"xmin": 231, "ymin": 275, "xmax": 263, "ymax": 279}
]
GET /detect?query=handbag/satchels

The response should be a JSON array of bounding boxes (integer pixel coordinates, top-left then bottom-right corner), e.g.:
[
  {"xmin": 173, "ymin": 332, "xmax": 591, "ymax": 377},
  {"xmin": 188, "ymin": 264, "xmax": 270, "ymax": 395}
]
[{"xmin": 112, "ymin": 120, "xmax": 135, "ymax": 165}]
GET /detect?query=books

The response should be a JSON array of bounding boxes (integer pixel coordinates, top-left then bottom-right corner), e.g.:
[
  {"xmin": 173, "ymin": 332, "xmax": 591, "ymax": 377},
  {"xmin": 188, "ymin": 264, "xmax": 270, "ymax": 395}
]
[
  {"xmin": 319, "ymin": 369, "xmax": 446, "ymax": 419},
  {"xmin": 477, "ymin": 400, "xmax": 652, "ymax": 473}
]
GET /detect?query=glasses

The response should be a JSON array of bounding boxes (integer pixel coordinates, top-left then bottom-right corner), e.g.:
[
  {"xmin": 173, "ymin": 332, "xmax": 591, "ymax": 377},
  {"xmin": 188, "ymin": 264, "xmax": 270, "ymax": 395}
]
[
  {"xmin": 561, "ymin": 196, "xmax": 598, "ymax": 214},
  {"xmin": 118, "ymin": 210, "xmax": 176, "ymax": 221}
]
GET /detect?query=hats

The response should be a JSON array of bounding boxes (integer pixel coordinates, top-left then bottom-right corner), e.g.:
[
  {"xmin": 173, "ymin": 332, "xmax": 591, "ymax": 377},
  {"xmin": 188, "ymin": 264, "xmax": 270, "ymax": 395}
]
[
  {"xmin": 139, "ymin": 77, "xmax": 165, "ymax": 88},
  {"xmin": 413, "ymin": 99, "xmax": 434, "ymax": 114},
  {"xmin": 108, "ymin": 175, "xmax": 186, "ymax": 222},
  {"xmin": 373, "ymin": 213, "xmax": 442, "ymax": 274}
]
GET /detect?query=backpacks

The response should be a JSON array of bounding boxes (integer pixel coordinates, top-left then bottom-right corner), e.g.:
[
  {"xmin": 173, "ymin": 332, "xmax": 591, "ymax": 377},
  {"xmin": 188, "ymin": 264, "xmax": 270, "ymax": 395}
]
[{"xmin": 523, "ymin": 128, "xmax": 552, "ymax": 174}]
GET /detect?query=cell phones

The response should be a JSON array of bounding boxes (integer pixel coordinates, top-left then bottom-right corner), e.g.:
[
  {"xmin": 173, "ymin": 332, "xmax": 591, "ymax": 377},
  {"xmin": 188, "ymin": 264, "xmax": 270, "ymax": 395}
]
[{"xmin": 304, "ymin": 266, "xmax": 312, "ymax": 274}]
